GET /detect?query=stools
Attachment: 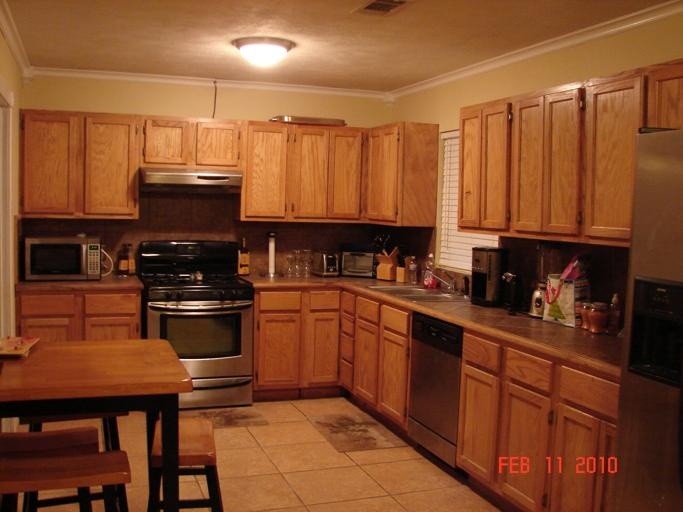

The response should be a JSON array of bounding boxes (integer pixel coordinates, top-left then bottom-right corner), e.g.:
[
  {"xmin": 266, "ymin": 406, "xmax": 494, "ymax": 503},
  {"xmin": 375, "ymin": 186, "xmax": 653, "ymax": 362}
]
[
  {"xmin": 1, "ymin": 428, "xmax": 99, "ymax": 459},
  {"xmin": 19, "ymin": 409, "xmax": 131, "ymax": 512},
  {"xmin": 151, "ymin": 419, "xmax": 223, "ymax": 512},
  {"xmin": 0, "ymin": 452, "xmax": 130, "ymax": 512}
]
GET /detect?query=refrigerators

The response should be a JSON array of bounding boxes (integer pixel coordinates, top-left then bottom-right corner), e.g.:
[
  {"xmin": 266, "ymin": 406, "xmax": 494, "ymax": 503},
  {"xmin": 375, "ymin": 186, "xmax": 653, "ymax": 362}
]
[{"xmin": 608, "ymin": 126, "xmax": 683, "ymax": 507}]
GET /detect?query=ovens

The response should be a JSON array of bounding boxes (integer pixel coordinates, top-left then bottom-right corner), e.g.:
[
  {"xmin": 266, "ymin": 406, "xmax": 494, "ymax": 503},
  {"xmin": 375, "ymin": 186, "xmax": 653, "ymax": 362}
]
[{"xmin": 146, "ymin": 300, "xmax": 254, "ymax": 409}]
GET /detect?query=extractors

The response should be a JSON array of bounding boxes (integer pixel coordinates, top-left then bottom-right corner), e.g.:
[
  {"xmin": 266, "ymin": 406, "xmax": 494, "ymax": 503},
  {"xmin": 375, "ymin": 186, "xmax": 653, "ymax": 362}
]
[{"xmin": 136, "ymin": 164, "xmax": 242, "ymax": 196}]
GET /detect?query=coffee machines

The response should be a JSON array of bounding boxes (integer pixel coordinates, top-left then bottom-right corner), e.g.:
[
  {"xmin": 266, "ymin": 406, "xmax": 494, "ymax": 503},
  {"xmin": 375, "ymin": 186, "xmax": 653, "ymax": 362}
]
[{"xmin": 470, "ymin": 245, "xmax": 507, "ymax": 306}]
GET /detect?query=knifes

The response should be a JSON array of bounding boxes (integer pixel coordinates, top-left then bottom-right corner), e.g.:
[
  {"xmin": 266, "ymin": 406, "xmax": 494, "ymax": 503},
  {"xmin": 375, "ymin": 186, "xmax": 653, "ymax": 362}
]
[{"xmin": 370, "ymin": 232, "xmax": 394, "ymax": 254}]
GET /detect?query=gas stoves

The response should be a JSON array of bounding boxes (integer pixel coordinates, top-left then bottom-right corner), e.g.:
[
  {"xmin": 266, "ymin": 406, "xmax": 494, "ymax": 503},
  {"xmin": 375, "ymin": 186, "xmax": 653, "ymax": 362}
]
[{"xmin": 138, "ymin": 270, "xmax": 254, "ymax": 300}]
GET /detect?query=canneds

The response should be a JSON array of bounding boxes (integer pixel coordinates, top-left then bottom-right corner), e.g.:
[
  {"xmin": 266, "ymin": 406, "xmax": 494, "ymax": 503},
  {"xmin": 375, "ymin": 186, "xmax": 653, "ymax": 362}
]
[{"xmin": 581, "ymin": 302, "xmax": 609, "ymax": 334}]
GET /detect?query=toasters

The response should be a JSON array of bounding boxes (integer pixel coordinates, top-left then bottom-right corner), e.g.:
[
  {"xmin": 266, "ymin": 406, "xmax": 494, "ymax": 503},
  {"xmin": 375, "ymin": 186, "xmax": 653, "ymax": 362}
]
[{"xmin": 311, "ymin": 251, "xmax": 339, "ymax": 277}]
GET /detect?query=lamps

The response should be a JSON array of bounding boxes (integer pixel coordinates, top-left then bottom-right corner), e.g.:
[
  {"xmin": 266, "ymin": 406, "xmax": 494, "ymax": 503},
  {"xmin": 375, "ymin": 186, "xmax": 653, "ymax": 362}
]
[{"xmin": 234, "ymin": 37, "xmax": 293, "ymax": 68}]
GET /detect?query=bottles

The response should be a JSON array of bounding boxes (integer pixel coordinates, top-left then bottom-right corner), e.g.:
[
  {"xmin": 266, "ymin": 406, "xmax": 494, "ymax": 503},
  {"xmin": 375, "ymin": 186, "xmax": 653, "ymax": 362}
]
[
  {"xmin": 116, "ymin": 241, "xmax": 136, "ymax": 280},
  {"xmin": 422, "ymin": 254, "xmax": 436, "ymax": 289},
  {"xmin": 580, "ymin": 302, "xmax": 607, "ymax": 335},
  {"xmin": 609, "ymin": 291, "xmax": 620, "ymax": 334},
  {"xmin": 405, "ymin": 254, "xmax": 417, "ymax": 284},
  {"xmin": 235, "ymin": 235, "xmax": 250, "ymax": 276}
]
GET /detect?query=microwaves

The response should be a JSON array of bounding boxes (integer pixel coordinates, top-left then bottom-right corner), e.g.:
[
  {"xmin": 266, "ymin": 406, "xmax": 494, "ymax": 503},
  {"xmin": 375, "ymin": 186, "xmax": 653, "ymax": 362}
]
[{"xmin": 22, "ymin": 234, "xmax": 101, "ymax": 281}]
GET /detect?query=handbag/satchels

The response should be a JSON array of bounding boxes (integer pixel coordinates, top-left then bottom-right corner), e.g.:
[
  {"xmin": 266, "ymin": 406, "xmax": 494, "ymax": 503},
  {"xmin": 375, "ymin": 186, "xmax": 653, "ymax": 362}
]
[{"xmin": 543, "ymin": 274, "xmax": 590, "ymax": 328}]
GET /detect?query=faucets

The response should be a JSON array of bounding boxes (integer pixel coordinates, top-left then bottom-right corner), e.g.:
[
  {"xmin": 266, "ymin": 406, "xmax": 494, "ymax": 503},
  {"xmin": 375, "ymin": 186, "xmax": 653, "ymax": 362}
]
[{"xmin": 425, "ymin": 268, "xmax": 456, "ymax": 292}]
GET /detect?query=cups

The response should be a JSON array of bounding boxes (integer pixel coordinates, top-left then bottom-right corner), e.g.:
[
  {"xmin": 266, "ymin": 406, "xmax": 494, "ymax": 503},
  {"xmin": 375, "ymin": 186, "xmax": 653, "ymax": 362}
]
[{"xmin": 286, "ymin": 248, "xmax": 312, "ymax": 277}]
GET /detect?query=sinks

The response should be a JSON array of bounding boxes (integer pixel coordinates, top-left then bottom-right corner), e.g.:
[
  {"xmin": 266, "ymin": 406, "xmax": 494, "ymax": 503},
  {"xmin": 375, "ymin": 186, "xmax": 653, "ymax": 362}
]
[
  {"xmin": 370, "ymin": 286, "xmax": 425, "ymax": 291},
  {"xmin": 397, "ymin": 294, "xmax": 465, "ymax": 302}
]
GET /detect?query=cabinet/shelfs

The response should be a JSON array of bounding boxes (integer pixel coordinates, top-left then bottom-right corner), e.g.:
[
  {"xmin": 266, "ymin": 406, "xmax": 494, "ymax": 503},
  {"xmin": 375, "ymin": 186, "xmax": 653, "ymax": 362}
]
[
  {"xmin": 337, "ymin": 292, "xmax": 353, "ymax": 392},
  {"xmin": 367, "ymin": 124, "xmax": 437, "ymax": 228},
  {"xmin": 352, "ymin": 294, "xmax": 410, "ymax": 427},
  {"xmin": 140, "ymin": 116, "xmax": 240, "ymax": 171},
  {"xmin": 549, "ymin": 362, "xmax": 620, "ymax": 511},
  {"xmin": 17, "ymin": 291, "xmax": 78, "ymax": 342},
  {"xmin": 455, "ymin": 329, "xmax": 549, "ymax": 512},
  {"xmin": 455, "ymin": 101, "xmax": 509, "ymax": 232},
  {"xmin": 583, "ymin": 58, "xmax": 681, "ymax": 246},
  {"xmin": 293, "ymin": 126, "xmax": 366, "ymax": 221},
  {"xmin": 240, "ymin": 124, "xmax": 291, "ymax": 219},
  {"xmin": 254, "ymin": 291, "xmax": 337, "ymax": 391},
  {"xmin": 510, "ymin": 86, "xmax": 584, "ymax": 238},
  {"xmin": 78, "ymin": 289, "xmax": 138, "ymax": 340},
  {"xmin": 18, "ymin": 109, "xmax": 139, "ymax": 221}
]
